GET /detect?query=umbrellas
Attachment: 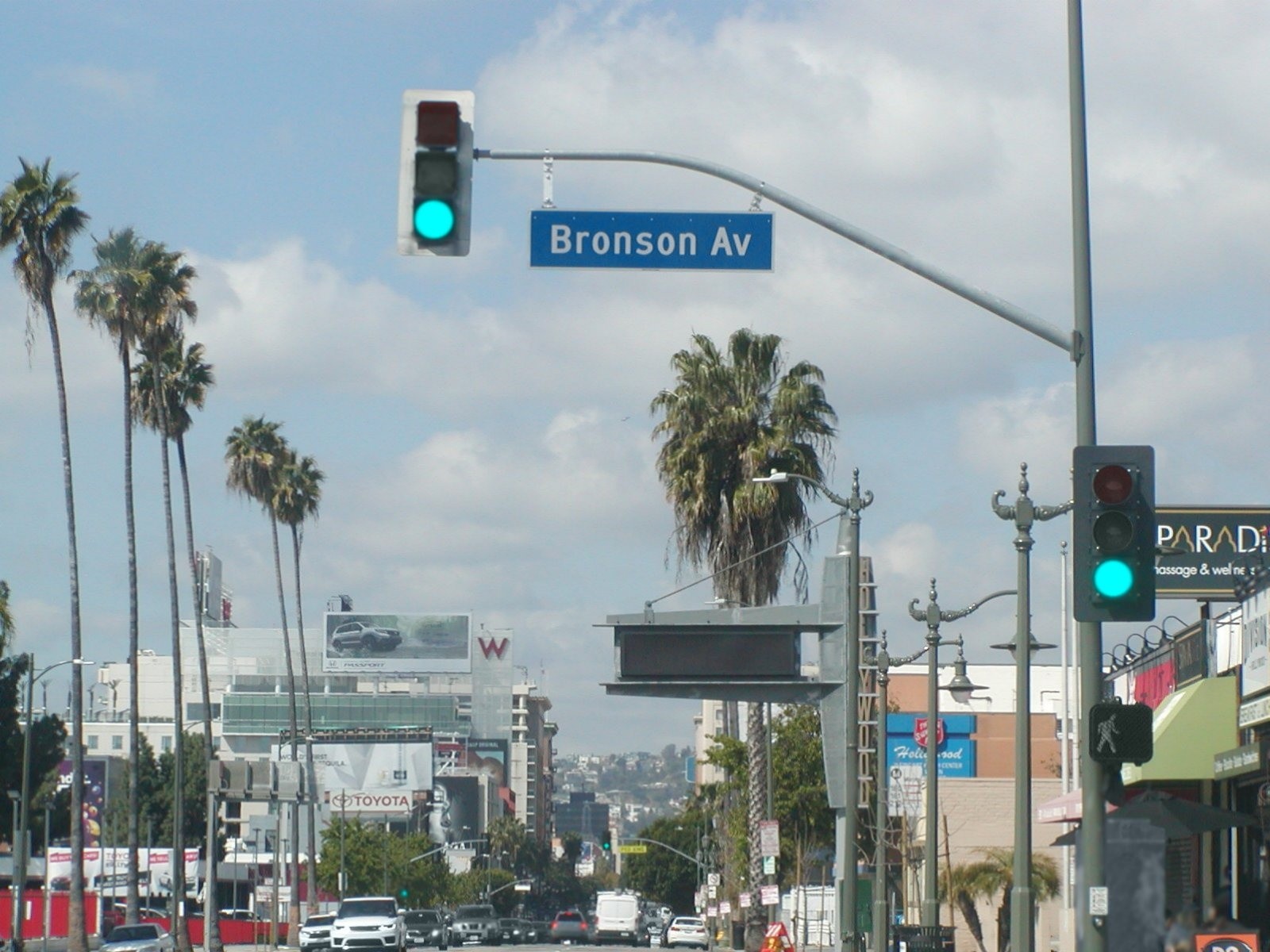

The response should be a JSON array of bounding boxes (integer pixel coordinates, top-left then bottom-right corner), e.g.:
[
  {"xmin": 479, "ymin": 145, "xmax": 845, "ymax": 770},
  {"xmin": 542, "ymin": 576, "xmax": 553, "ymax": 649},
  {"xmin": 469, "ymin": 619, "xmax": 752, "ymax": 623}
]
[{"xmin": 1049, "ymin": 788, "xmax": 1255, "ymax": 846}]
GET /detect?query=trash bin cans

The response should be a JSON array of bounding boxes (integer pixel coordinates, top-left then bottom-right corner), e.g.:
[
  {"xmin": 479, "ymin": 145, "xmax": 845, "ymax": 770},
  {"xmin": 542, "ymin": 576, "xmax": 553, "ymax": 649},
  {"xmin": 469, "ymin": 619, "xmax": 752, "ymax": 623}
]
[
  {"xmin": 902, "ymin": 926, "xmax": 957, "ymax": 952},
  {"xmin": 732, "ymin": 920, "xmax": 745, "ymax": 950}
]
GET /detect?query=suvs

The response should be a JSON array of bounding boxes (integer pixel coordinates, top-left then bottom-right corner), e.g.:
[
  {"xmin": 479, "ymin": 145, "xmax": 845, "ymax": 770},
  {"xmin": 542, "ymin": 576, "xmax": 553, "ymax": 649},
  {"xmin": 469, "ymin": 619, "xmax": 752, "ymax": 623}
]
[
  {"xmin": 330, "ymin": 897, "xmax": 408, "ymax": 952},
  {"xmin": 448, "ymin": 904, "xmax": 502, "ymax": 948},
  {"xmin": 331, "ymin": 621, "xmax": 403, "ymax": 653}
]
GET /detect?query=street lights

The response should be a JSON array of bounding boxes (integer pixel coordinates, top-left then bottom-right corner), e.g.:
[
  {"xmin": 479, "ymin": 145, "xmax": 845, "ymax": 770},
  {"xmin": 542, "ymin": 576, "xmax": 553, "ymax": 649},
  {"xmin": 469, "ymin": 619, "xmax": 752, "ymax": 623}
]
[
  {"xmin": 992, "ymin": 461, "xmax": 1190, "ymax": 952},
  {"xmin": 906, "ymin": 579, "xmax": 1062, "ymax": 952},
  {"xmin": 41, "ymin": 800, "xmax": 55, "ymax": 937},
  {"xmin": 169, "ymin": 718, "xmax": 409, "ymax": 948},
  {"xmin": 751, "ymin": 464, "xmax": 866, "ymax": 952},
  {"xmin": 864, "ymin": 627, "xmax": 990, "ymax": 951},
  {"xmin": 7, "ymin": 789, "xmax": 21, "ymax": 915},
  {"xmin": 9, "ymin": 652, "xmax": 97, "ymax": 952},
  {"xmin": 704, "ymin": 818, "xmax": 712, "ymax": 884},
  {"xmin": 695, "ymin": 825, "xmax": 703, "ymax": 893},
  {"xmin": 706, "ymin": 597, "xmax": 777, "ymax": 930}
]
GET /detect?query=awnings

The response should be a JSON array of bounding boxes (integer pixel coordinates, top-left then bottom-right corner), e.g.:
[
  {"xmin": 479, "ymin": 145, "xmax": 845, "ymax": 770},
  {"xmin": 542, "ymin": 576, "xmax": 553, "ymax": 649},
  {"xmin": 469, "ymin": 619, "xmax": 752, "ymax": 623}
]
[{"xmin": 1119, "ymin": 674, "xmax": 1239, "ymax": 786}]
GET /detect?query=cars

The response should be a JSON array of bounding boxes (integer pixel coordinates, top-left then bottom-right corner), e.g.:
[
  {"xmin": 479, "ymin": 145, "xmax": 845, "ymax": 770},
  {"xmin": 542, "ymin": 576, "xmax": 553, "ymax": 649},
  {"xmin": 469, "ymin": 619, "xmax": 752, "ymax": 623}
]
[
  {"xmin": 51, "ymin": 871, "xmax": 90, "ymax": 891},
  {"xmin": 218, "ymin": 908, "xmax": 264, "ymax": 922},
  {"xmin": 161, "ymin": 871, "xmax": 197, "ymax": 894},
  {"xmin": 637, "ymin": 917, "xmax": 652, "ymax": 948},
  {"xmin": 404, "ymin": 908, "xmax": 451, "ymax": 951},
  {"xmin": 114, "ymin": 904, "xmax": 165, "ymax": 920},
  {"xmin": 501, "ymin": 910, "xmax": 589, "ymax": 945},
  {"xmin": 667, "ymin": 916, "xmax": 710, "ymax": 951},
  {"xmin": 297, "ymin": 911, "xmax": 338, "ymax": 952},
  {"xmin": 98, "ymin": 921, "xmax": 175, "ymax": 952}
]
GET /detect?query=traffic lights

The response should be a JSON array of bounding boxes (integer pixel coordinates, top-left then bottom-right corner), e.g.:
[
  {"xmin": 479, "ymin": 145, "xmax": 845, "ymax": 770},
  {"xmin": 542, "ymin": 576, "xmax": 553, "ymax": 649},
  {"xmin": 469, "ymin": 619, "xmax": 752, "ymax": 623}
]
[
  {"xmin": 400, "ymin": 886, "xmax": 409, "ymax": 900},
  {"xmin": 1087, "ymin": 699, "xmax": 1156, "ymax": 768},
  {"xmin": 1070, "ymin": 444, "xmax": 1157, "ymax": 623},
  {"xmin": 395, "ymin": 90, "xmax": 475, "ymax": 262},
  {"xmin": 602, "ymin": 830, "xmax": 612, "ymax": 851}
]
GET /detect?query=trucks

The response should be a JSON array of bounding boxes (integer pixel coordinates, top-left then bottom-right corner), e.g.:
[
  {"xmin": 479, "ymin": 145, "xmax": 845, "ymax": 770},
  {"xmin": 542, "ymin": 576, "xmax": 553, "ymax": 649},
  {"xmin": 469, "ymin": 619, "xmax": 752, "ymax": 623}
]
[{"xmin": 592, "ymin": 892, "xmax": 639, "ymax": 948}]
